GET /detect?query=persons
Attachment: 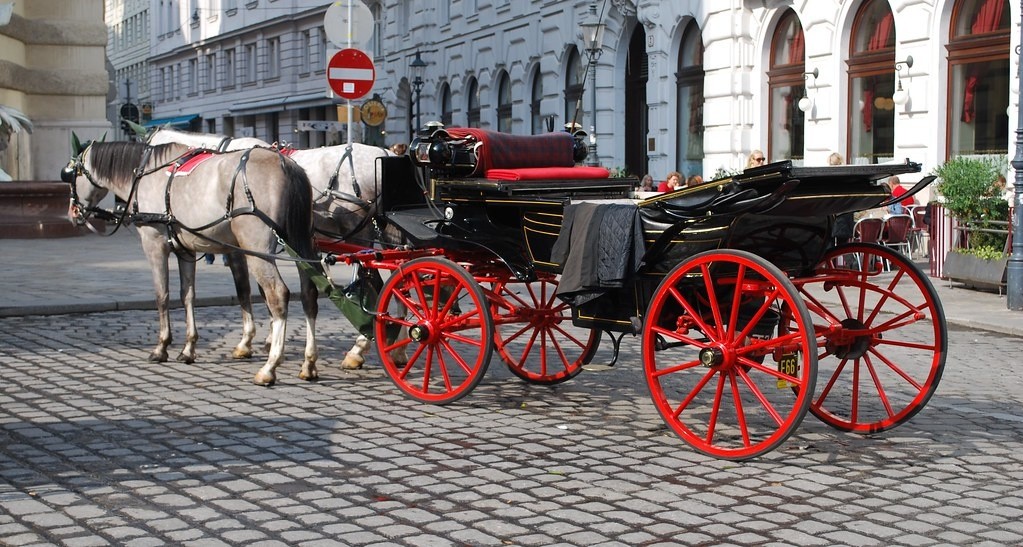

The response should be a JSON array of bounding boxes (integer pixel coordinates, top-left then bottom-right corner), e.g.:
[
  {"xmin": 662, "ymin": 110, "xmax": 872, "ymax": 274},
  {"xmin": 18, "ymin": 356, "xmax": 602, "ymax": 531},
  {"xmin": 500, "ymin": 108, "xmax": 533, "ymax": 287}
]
[
  {"xmin": 879, "ymin": 183, "xmax": 901, "ymax": 270},
  {"xmin": 658, "ymin": 173, "xmax": 682, "ymax": 192},
  {"xmin": 686, "ymin": 175, "xmax": 703, "ymax": 187},
  {"xmin": 642, "ymin": 174, "xmax": 658, "ymax": 192},
  {"xmin": 888, "ymin": 176, "xmax": 914, "ymax": 206},
  {"xmin": 829, "ymin": 153, "xmax": 856, "ymax": 269},
  {"xmin": 747, "ymin": 150, "xmax": 765, "ymax": 168},
  {"xmin": 992, "ymin": 174, "xmax": 1015, "ymax": 207},
  {"xmin": 389, "ymin": 144, "xmax": 407, "ymax": 155}
]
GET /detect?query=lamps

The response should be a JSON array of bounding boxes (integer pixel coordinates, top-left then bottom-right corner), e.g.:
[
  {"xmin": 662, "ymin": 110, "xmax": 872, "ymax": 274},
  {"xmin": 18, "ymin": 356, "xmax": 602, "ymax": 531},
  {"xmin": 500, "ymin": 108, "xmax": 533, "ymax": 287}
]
[
  {"xmin": 192, "ymin": 9, "xmax": 199, "ymax": 24},
  {"xmin": 798, "ymin": 68, "xmax": 819, "ymax": 111},
  {"xmin": 893, "ymin": 56, "xmax": 913, "ymax": 105}
]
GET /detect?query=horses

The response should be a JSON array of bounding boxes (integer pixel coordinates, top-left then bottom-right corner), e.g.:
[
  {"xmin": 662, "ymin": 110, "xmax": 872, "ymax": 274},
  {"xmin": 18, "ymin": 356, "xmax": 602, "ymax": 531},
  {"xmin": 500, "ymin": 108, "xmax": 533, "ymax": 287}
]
[
  {"xmin": 124, "ymin": 119, "xmax": 410, "ymax": 370},
  {"xmin": 63, "ymin": 129, "xmax": 323, "ymax": 386}
]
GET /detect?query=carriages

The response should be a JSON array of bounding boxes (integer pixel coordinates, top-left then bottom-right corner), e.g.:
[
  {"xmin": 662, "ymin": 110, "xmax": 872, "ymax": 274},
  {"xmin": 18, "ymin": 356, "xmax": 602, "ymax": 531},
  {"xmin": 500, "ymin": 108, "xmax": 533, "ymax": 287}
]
[{"xmin": 62, "ymin": 117, "xmax": 949, "ymax": 460}]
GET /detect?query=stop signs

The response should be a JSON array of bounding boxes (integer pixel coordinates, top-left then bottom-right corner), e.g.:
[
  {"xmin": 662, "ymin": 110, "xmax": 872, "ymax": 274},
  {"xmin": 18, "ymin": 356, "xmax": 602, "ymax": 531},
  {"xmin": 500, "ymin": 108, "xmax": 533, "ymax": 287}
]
[{"xmin": 326, "ymin": 49, "xmax": 376, "ymax": 101}]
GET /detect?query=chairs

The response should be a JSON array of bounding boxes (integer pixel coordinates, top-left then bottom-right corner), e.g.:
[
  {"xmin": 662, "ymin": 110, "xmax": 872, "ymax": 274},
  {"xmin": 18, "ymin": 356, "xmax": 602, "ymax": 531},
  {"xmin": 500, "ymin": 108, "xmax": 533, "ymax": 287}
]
[
  {"xmin": 850, "ymin": 204, "xmax": 929, "ymax": 272},
  {"xmin": 445, "ymin": 126, "xmax": 609, "ymax": 181}
]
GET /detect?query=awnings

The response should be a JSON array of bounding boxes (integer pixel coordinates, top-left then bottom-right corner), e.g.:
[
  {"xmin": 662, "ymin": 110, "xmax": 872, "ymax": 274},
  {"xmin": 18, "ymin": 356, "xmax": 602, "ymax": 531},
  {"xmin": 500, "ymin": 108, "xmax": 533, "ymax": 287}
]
[{"xmin": 144, "ymin": 114, "xmax": 198, "ymax": 130}]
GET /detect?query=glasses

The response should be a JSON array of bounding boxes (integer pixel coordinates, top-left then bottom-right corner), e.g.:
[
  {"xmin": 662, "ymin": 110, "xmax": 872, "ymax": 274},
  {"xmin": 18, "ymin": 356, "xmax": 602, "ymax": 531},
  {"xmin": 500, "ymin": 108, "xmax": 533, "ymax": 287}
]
[{"xmin": 753, "ymin": 157, "xmax": 765, "ymax": 162}]
